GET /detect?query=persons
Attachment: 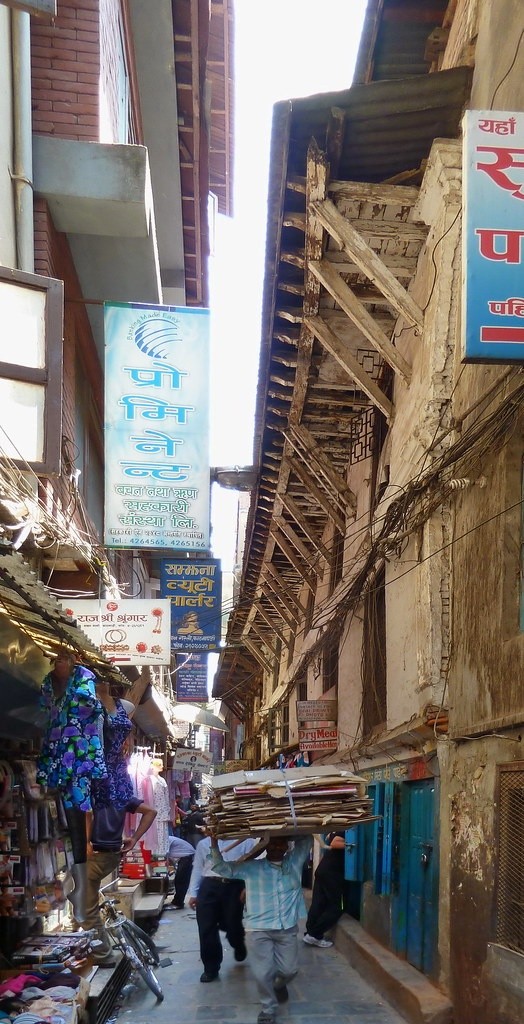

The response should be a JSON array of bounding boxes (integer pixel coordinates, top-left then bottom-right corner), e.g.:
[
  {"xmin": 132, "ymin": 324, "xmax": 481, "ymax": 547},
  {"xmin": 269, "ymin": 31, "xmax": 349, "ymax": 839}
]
[
  {"xmin": 82, "ymin": 795, "xmax": 158, "ymax": 969},
  {"xmin": 35, "ymin": 654, "xmax": 108, "ymax": 924},
  {"xmin": 185, "ymin": 835, "xmax": 256, "ymax": 983},
  {"xmin": 174, "ymin": 795, "xmax": 208, "ymax": 855},
  {"xmin": 141, "ymin": 759, "xmax": 170, "ymax": 857},
  {"xmin": 91, "ymin": 682, "xmax": 136, "ymax": 810},
  {"xmin": 303, "ymin": 831, "xmax": 345, "ymax": 948},
  {"xmin": 209, "ymin": 833, "xmax": 314, "ymax": 1024}
]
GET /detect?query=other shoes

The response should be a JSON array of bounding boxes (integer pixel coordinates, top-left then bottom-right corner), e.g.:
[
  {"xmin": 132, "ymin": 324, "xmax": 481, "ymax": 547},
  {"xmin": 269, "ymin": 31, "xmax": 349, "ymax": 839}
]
[
  {"xmin": 93, "ymin": 962, "xmax": 116, "ymax": 968},
  {"xmin": 164, "ymin": 903, "xmax": 184, "ymax": 910},
  {"xmin": 200, "ymin": 971, "xmax": 219, "ymax": 982},
  {"xmin": 234, "ymin": 943, "xmax": 247, "ymax": 961}
]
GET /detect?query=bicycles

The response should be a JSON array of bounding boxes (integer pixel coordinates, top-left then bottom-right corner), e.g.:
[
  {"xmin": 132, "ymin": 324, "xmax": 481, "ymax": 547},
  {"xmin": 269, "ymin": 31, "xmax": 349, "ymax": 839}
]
[{"xmin": 98, "ymin": 874, "xmax": 174, "ymax": 1001}]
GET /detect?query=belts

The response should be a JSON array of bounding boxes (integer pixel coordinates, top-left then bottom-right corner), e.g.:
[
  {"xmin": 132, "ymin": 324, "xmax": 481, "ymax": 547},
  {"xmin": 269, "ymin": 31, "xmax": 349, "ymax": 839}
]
[{"xmin": 93, "ymin": 845, "xmax": 120, "ymax": 852}]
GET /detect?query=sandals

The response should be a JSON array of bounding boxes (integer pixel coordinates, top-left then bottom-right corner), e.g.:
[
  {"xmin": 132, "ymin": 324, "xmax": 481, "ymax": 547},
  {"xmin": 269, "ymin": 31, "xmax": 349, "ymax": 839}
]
[
  {"xmin": 257, "ymin": 1011, "xmax": 275, "ymax": 1024},
  {"xmin": 275, "ymin": 987, "xmax": 288, "ymax": 1002}
]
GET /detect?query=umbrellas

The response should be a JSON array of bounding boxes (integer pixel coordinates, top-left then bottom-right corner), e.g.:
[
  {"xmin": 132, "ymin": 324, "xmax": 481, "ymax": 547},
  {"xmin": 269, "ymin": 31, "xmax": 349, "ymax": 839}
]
[{"xmin": 172, "ymin": 703, "xmax": 231, "ymax": 746}]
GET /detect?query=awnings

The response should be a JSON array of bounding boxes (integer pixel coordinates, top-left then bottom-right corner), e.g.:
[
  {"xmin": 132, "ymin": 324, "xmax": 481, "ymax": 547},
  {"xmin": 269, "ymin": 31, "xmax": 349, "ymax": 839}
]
[{"xmin": 0, "ymin": 529, "xmax": 132, "ymax": 687}]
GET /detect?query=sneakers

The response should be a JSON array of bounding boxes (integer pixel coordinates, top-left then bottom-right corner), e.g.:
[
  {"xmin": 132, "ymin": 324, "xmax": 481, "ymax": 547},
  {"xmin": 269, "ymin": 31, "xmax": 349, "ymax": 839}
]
[{"xmin": 303, "ymin": 934, "xmax": 333, "ymax": 948}]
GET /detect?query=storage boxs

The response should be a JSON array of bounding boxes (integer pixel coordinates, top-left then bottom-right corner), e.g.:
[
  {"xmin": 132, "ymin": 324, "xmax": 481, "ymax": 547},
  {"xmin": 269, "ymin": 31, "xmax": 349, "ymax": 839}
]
[{"xmin": 123, "ymin": 851, "xmax": 169, "ymax": 878}]
